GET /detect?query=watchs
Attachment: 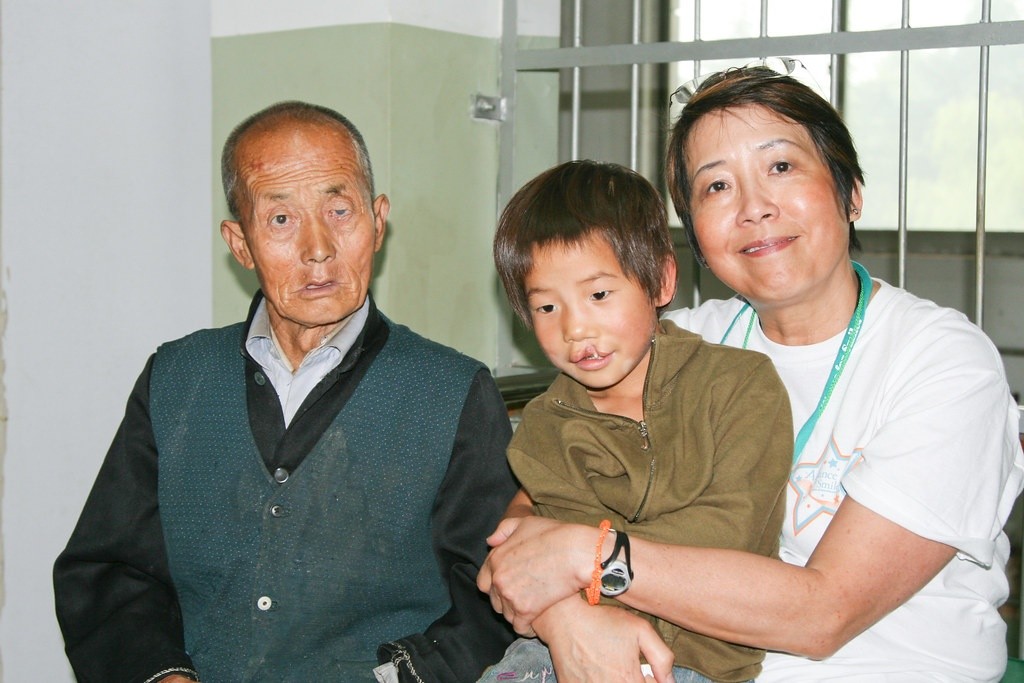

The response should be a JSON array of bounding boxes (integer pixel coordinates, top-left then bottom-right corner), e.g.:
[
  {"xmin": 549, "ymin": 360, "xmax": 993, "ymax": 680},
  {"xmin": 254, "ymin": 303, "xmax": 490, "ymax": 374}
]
[{"xmin": 599, "ymin": 529, "xmax": 634, "ymax": 597}]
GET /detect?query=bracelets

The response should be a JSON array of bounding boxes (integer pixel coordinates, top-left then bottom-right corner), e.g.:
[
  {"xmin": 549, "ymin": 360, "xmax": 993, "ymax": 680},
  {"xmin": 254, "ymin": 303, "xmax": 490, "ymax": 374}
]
[{"xmin": 584, "ymin": 519, "xmax": 610, "ymax": 605}]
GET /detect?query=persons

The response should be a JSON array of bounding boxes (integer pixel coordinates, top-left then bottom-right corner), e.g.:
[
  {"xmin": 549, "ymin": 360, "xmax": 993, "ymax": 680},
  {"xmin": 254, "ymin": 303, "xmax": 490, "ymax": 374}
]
[
  {"xmin": 469, "ymin": 159, "xmax": 792, "ymax": 683},
  {"xmin": 476, "ymin": 68, "xmax": 1024, "ymax": 683},
  {"xmin": 53, "ymin": 100, "xmax": 526, "ymax": 683}
]
